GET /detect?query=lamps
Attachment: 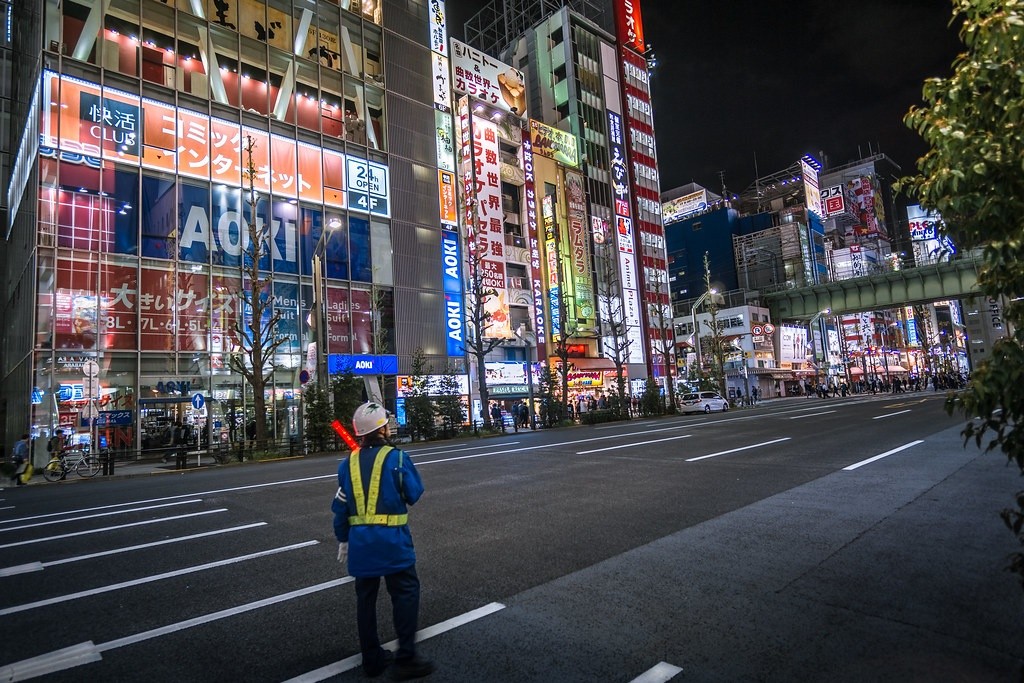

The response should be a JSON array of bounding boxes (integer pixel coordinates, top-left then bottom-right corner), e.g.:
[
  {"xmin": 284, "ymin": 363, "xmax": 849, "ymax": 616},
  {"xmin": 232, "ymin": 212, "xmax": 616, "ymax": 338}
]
[
  {"xmin": 472, "ymin": 103, "xmax": 485, "ymax": 115},
  {"xmin": 572, "ymin": 154, "xmax": 590, "ymax": 168},
  {"xmin": 489, "ymin": 111, "xmax": 502, "ymax": 120}
]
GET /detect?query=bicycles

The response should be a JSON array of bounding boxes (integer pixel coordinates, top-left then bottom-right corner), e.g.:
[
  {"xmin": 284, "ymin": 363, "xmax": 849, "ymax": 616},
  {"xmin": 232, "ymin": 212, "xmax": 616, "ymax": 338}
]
[{"xmin": 43, "ymin": 450, "xmax": 101, "ymax": 482}]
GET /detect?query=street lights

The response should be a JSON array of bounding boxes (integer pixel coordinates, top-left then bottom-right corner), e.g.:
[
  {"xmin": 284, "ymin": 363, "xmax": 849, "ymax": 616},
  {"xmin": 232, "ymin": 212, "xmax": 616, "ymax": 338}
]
[
  {"xmin": 809, "ymin": 310, "xmax": 830, "ymax": 397},
  {"xmin": 692, "ymin": 288, "xmax": 717, "ymax": 391},
  {"xmin": 310, "ymin": 217, "xmax": 343, "ymax": 449},
  {"xmin": 930, "ymin": 331, "xmax": 944, "ymax": 387},
  {"xmin": 879, "ymin": 323, "xmax": 897, "ymax": 390}
]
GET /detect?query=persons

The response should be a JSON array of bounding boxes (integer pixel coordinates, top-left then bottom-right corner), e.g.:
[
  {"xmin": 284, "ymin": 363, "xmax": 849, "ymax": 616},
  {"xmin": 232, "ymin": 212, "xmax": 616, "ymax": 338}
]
[
  {"xmin": 729, "ymin": 370, "xmax": 972, "ymax": 405},
  {"xmin": 479, "ymin": 389, "xmax": 645, "ymax": 434},
  {"xmin": 331, "ymin": 402, "xmax": 437, "ymax": 676},
  {"xmin": 158, "ymin": 416, "xmax": 260, "ymax": 464},
  {"xmin": 11, "ymin": 429, "xmax": 69, "ymax": 482}
]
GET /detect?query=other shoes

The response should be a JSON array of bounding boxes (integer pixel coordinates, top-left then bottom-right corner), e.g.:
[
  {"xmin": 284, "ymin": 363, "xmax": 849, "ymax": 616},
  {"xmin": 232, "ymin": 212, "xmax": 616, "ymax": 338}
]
[
  {"xmin": 396, "ymin": 654, "xmax": 437, "ymax": 680},
  {"xmin": 365, "ymin": 650, "xmax": 392, "ymax": 676}
]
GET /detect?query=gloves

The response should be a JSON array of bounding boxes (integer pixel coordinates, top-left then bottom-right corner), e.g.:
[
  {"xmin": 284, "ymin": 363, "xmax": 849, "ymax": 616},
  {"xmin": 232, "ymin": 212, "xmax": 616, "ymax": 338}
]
[{"xmin": 337, "ymin": 542, "xmax": 348, "ymax": 564}]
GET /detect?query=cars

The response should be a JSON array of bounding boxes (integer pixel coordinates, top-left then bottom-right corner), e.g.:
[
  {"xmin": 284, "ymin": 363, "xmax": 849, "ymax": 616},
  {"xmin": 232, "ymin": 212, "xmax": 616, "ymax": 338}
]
[{"xmin": 679, "ymin": 390, "xmax": 730, "ymax": 415}]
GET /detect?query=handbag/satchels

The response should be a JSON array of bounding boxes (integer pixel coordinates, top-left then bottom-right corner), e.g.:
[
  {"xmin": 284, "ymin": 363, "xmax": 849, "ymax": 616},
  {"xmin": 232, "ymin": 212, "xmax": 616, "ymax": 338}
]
[
  {"xmin": 12, "ymin": 455, "xmax": 23, "ymax": 463},
  {"xmin": 15, "ymin": 462, "xmax": 32, "ymax": 482}
]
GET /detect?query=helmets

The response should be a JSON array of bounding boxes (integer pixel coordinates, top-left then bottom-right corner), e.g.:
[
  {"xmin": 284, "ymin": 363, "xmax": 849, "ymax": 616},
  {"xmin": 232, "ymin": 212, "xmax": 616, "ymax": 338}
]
[{"xmin": 353, "ymin": 402, "xmax": 391, "ymax": 436}]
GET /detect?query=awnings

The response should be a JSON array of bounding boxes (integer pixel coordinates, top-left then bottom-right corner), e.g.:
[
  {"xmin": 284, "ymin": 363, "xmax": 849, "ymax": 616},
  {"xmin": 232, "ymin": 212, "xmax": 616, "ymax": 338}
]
[{"xmin": 565, "ymin": 356, "xmax": 615, "ymax": 372}]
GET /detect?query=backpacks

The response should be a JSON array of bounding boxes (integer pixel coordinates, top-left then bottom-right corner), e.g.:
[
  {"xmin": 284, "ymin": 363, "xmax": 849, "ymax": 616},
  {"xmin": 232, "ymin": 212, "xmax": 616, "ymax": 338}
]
[{"xmin": 47, "ymin": 436, "xmax": 60, "ymax": 451}]
